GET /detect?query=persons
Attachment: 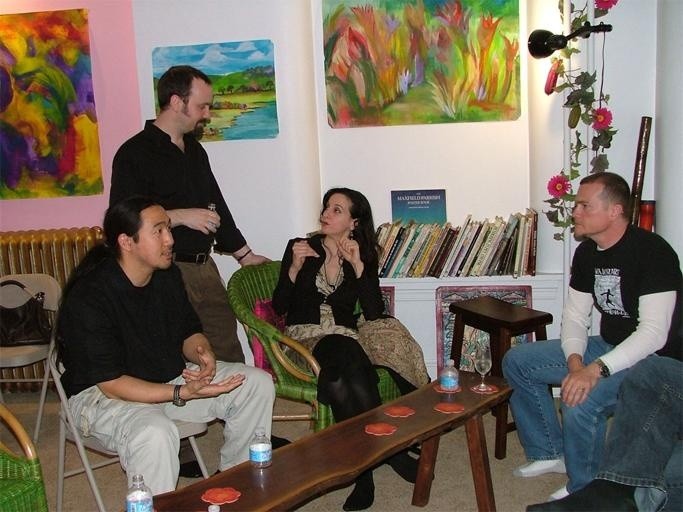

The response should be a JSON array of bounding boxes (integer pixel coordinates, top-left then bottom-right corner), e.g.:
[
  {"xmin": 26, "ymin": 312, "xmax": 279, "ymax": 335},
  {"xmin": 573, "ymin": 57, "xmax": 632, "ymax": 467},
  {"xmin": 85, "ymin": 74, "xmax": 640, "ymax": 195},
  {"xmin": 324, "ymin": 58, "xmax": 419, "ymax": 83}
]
[
  {"xmin": 525, "ymin": 356, "xmax": 682, "ymax": 511},
  {"xmin": 498, "ymin": 170, "xmax": 682, "ymax": 502},
  {"xmin": 273, "ymin": 187, "xmax": 434, "ymax": 511},
  {"xmin": 54, "ymin": 196, "xmax": 276, "ymax": 497},
  {"xmin": 110, "ymin": 66, "xmax": 270, "ymax": 363}
]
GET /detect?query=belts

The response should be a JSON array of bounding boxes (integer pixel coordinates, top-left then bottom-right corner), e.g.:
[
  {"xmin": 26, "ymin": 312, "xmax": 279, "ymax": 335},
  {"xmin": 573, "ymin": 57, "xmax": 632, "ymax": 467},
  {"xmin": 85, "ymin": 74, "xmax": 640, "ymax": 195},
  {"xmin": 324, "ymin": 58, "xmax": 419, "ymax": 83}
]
[{"xmin": 173, "ymin": 251, "xmax": 210, "ymax": 265}]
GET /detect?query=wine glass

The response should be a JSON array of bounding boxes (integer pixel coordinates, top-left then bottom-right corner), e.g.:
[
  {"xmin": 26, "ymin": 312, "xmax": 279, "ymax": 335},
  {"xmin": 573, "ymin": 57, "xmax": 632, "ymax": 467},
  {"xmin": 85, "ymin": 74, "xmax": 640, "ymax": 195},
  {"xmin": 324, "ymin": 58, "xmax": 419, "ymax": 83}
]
[{"xmin": 474, "ymin": 346, "xmax": 492, "ymax": 391}]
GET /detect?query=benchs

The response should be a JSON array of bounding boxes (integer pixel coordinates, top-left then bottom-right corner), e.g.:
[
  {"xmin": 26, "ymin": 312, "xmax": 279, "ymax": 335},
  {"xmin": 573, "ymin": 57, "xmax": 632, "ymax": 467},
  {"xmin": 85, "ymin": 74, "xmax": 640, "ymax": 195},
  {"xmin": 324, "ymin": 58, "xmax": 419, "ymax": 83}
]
[{"xmin": 116, "ymin": 372, "xmax": 514, "ymax": 512}]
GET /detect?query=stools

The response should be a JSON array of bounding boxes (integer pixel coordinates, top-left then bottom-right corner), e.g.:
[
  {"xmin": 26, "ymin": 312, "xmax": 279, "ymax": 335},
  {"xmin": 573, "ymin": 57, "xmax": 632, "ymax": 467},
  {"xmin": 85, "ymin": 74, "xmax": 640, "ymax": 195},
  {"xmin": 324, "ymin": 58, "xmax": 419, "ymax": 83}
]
[{"xmin": 448, "ymin": 297, "xmax": 555, "ymax": 460}]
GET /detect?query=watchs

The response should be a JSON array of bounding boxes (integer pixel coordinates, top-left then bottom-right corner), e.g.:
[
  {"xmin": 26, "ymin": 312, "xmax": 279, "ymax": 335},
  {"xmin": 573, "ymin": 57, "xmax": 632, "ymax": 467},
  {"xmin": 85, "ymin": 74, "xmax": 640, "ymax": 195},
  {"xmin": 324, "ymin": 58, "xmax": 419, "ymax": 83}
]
[
  {"xmin": 594, "ymin": 357, "xmax": 610, "ymax": 378},
  {"xmin": 171, "ymin": 384, "xmax": 186, "ymax": 407}
]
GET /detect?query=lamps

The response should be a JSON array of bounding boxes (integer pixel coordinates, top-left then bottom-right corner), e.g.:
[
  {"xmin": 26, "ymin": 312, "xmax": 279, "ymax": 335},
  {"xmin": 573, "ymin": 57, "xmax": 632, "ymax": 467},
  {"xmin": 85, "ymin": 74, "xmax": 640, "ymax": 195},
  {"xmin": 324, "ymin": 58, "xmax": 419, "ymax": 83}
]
[{"xmin": 526, "ymin": 20, "xmax": 614, "ymax": 61}]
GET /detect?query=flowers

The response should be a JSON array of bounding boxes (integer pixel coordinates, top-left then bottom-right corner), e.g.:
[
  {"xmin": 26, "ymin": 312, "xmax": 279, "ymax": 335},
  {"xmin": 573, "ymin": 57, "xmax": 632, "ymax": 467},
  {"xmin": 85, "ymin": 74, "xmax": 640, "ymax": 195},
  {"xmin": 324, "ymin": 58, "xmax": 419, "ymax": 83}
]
[{"xmin": 539, "ymin": 0, "xmax": 621, "ymax": 242}]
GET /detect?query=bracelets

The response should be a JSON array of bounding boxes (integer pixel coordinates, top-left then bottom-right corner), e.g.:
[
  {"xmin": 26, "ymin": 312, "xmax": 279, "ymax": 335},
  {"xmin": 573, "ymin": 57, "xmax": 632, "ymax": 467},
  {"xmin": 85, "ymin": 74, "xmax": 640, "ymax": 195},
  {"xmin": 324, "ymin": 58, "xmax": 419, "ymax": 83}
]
[{"xmin": 236, "ymin": 250, "xmax": 253, "ymax": 260}]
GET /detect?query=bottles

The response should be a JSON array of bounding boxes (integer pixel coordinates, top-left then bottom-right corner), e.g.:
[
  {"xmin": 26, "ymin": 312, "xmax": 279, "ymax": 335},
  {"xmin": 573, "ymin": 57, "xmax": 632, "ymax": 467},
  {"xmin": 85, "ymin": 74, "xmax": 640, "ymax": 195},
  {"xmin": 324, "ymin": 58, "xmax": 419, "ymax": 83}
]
[
  {"xmin": 126, "ymin": 476, "xmax": 153, "ymax": 512},
  {"xmin": 204, "ymin": 203, "xmax": 217, "ymax": 237},
  {"xmin": 249, "ymin": 431, "xmax": 275, "ymax": 491},
  {"xmin": 206, "ymin": 504, "xmax": 219, "ymax": 512},
  {"xmin": 440, "ymin": 358, "xmax": 459, "ymax": 413}
]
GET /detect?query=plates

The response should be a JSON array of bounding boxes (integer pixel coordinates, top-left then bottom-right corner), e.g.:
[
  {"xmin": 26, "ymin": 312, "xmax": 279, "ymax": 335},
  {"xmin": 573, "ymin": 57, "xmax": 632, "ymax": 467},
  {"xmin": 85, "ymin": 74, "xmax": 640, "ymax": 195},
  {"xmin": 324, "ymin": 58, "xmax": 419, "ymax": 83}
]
[
  {"xmin": 203, "ymin": 485, "xmax": 240, "ymax": 504},
  {"xmin": 383, "ymin": 406, "xmax": 414, "ymax": 419},
  {"xmin": 432, "ymin": 403, "xmax": 465, "ymax": 414},
  {"xmin": 366, "ymin": 421, "xmax": 395, "ymax": 435},
  {"xmin": 470, "ymin": 385, "xmax": 497, "ymax": 395},
  {"xmin": 433, "ymin": 383, "xmax": 462, "ymax": 393}
]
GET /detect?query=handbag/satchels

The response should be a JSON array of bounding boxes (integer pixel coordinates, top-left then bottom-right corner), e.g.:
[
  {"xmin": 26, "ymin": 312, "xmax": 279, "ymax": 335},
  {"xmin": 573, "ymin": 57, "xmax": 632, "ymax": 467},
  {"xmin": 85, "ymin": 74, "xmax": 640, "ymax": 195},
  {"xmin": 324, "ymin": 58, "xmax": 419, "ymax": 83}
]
[{"xmin": 0, "ymin": 280, "xmax": 50, "ymax": 347}]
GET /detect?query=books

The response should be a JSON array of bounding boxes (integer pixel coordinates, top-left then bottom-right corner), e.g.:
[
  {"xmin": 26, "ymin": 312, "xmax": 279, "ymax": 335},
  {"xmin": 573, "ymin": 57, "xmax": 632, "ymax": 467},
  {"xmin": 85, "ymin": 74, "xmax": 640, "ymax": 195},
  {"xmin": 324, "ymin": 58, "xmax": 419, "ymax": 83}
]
[{"xmin": 369, "ymin": 207, "xmax": 537, "ymax": 280}]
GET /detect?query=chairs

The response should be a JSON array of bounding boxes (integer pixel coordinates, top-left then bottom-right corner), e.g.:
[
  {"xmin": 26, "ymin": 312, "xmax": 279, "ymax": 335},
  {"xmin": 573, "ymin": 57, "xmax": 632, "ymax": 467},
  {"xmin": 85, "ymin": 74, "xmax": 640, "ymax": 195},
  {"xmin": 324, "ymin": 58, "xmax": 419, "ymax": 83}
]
[{"xmin": 228, "ymin": 259, "xmax": 341, "ymax": 437}]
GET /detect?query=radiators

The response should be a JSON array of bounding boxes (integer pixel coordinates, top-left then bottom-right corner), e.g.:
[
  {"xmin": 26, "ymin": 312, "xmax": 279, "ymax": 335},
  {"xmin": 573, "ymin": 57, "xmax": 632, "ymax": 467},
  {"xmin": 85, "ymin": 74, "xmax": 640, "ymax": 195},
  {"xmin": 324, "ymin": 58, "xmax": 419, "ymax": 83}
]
[{"xmin": 0, "ymin": 223, "xmax": 104, "ymax": 396}]
